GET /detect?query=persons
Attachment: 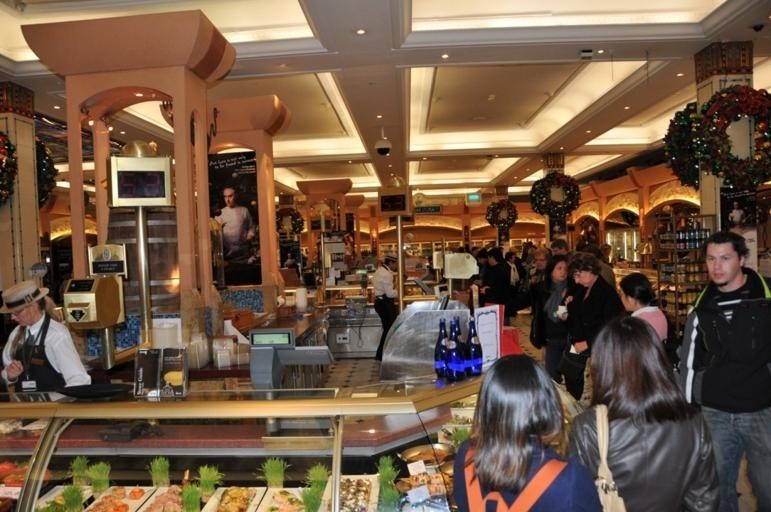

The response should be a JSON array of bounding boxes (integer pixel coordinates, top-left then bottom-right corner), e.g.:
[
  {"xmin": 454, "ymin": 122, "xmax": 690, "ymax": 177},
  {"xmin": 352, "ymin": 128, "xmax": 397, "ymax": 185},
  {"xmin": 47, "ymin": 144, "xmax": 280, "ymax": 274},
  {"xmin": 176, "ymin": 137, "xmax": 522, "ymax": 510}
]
[
  {"xmin": 729, "ymin": 202, "xmax": 746, "ymax": 228},
  {"xmin": 534, "ymin": 253, "xmax": 584, "ymax": 401},
  {"xmin": 365, "ymin": 254, "xmax": 399, "ymax": 362},
  {"xmin": 1, "ymin": 278, "xmax": 92, "ymax": 467},
  {"xmin": 678, "ymin": 230, "xmax": 771, "ymax": 512},
  {"xmin": 617, "ymin": 271, "xmax": 669, "ymax": 343},
  {"xmin": 453, "ymin": 350, "xmax": 605, "ymax": 511},
  {"xmin": 212, "ymin": 185, "xmax": 256, "ymax": 265},
  {"xmin": 564, "ymin": 250, "xmax": 624, "ymax": 401},
  {"xmin": 453, "ymin": 238, "xmax": 618, "ymax": 325},
  {"xmin": 570, "ymin": 317, "xmax": 721, "ymax": 512}
]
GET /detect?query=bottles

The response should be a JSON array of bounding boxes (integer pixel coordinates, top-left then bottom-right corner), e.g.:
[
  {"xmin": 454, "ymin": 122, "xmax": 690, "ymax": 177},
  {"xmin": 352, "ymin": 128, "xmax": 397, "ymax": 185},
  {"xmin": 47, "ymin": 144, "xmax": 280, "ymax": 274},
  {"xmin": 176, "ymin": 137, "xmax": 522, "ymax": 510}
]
[
  {"xmin": 435, "ymin": 315, "xmax": 449, "ymax": 382},
  {"xmin": 658, "ymin": 228, "xmax": 712, "ymax": 327},
  {"xmin": 446, "ymin": 322, "xmax": 467, "ymax": 381},
  {"xmin": 451, "ymin": 315, "xmax": 466, "ymax": 357},
  {"xmin": 464, "ymin": 317, "xmax": 483, "ymax": 378}
]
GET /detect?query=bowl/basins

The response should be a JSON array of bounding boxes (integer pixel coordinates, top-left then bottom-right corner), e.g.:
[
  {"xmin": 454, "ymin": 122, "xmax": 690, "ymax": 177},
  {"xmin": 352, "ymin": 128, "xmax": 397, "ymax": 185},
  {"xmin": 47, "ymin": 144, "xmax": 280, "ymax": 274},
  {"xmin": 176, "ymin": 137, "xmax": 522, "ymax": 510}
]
[{"xmin": 398, "ymin": 441, "xmax": 455, "ymax": 465}]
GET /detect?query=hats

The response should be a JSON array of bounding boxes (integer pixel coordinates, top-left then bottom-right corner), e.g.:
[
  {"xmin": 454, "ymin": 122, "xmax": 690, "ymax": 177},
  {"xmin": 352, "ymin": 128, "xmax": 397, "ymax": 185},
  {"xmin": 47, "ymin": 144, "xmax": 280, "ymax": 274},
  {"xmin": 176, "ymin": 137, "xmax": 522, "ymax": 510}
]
[
  {"xmin": 385, "ymin": 254, "xmax": 399, "ymax": 262},
  {"xmin": 0, "ymin": 281, "xmax": 49, "ymax": 314}
]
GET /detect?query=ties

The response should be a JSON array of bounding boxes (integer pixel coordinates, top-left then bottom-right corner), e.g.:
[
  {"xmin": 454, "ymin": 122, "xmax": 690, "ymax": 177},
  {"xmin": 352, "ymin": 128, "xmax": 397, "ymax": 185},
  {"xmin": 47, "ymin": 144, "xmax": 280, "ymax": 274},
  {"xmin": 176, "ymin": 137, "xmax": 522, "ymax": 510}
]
[{"xmin": 26, "ymin": 329, "xmax": 34, "ymax": 347}]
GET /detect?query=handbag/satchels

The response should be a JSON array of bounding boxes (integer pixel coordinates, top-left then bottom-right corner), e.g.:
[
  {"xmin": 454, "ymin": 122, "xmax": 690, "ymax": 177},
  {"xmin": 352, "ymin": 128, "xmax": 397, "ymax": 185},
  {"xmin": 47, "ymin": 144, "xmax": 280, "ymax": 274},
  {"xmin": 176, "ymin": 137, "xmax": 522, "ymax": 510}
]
[
  {"xmin": 595, "ymin": 476, "xmax": 627, "ymax": 511},
  {"xmin": 480, "ymin": 287, "xmax": 499, "ymax": 303},
  {"xmin": 557, "ymin": 342, "xmax": 591, "ymax": 384}
]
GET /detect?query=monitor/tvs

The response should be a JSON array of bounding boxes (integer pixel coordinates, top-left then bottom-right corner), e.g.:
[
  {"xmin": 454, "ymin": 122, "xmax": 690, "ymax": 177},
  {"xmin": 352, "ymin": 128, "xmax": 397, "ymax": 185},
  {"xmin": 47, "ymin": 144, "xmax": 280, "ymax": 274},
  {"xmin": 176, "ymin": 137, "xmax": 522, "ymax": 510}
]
[{"xmin": 439, "ymin": 294, "xmax": 450, "ymax": 310}]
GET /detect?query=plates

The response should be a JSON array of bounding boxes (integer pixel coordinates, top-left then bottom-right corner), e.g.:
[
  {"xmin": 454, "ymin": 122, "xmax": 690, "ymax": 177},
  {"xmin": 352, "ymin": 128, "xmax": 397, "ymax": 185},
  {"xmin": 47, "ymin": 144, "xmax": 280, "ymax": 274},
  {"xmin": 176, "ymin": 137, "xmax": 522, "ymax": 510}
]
[
  {"xmin": 314, "ymin": 473, "xmax": 379, "ymax": 512},
  {"xmin": 81, "ymin": 485, "xmax": 310, "ymax": 512},
  {"xmin": 36, "ymin": 483, "xmax": 94, "ymax": 511}
]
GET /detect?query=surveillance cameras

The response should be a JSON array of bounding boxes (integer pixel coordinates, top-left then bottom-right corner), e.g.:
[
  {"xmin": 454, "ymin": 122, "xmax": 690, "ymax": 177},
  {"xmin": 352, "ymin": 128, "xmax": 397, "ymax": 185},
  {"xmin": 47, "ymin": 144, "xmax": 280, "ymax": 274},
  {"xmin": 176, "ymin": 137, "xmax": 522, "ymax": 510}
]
[{"xmin": 375, "ymin": 127, "xmax": 392, "ymax": 156}]
